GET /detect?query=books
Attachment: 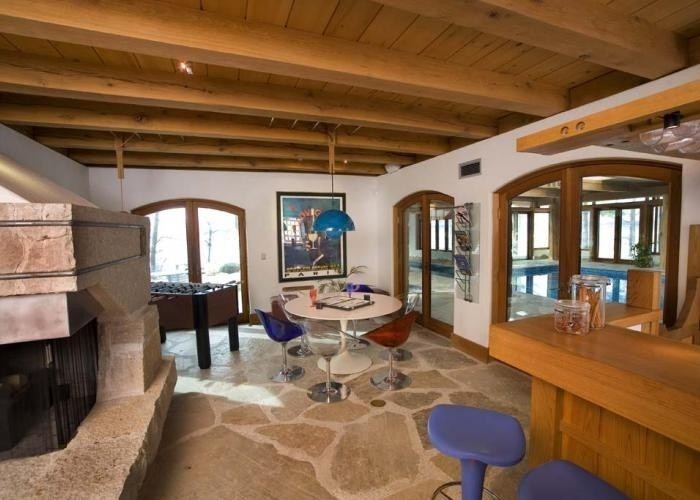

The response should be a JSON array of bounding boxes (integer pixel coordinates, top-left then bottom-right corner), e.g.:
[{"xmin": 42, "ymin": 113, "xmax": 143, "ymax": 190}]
[{"xmin": 454, "ymin": 207, "xmax": 469, "ymax": 271}]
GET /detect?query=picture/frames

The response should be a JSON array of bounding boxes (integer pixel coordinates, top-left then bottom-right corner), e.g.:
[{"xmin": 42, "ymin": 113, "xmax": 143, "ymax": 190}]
[{"xmin": 275, "ymin": 190, "xmax": 348, "ymax": 284}]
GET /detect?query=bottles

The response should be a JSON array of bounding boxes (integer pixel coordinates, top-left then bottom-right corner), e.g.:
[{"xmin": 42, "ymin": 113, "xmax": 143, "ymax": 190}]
[
  {"xmin": 553, "ymin": 299, "xmax": 592, "ymax": 337},
  {"xmin": 568, "ymin": 273, "xmax": 610, "ymax": 328}
]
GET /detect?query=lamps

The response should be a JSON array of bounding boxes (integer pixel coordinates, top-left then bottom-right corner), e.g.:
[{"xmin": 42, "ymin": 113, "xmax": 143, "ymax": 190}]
[{"xmin": 311, "ymin": 129, "xmax": 356, "ymax": 241}]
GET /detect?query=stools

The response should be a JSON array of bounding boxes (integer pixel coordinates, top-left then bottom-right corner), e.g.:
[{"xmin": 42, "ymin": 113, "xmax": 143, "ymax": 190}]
[
  {"xmin": 298, "ymin": 330, "xmax": 351, "ymax": 404},
  {"xmin": 379, "ymin": 290, "xmax": 419, "ymax": 363},
  {"xmin": 425, "ymin": 403, "xmax": 527, "ymax": 499},
  {"xmin": 274, "ymin": 284, "xmax": 314, "ymax": 360},
  {"xmin": 516, "ymin": 457, "xmax": 631, "ymax": 499},
  {"xmin": 338, "ymin": 281, "xmax": 374, "ymax": 351},
  {"xmin": 363, "ymin": 308, "xmax": 417, "ymax": 390},
  {"xmin": 253, "ymin": 308, "xmax": 308, "ymax": 386}
]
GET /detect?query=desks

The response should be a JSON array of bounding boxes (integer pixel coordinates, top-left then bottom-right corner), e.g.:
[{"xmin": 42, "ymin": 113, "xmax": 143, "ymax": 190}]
[{"xmin": 279, "ymin": 291, "xmax": 403, "ymax": 378}]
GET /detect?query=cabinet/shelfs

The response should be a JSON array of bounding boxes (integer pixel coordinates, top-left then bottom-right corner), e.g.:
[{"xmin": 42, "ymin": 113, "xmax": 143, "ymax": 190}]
[{"xmin": 452, "ymin": 202, "xmax": 474, "ymax": 303}]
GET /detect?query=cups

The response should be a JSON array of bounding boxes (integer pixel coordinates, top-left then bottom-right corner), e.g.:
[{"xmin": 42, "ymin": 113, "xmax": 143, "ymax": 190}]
[
  {"xmin": 310, "ymin": 289, "xmax": 317, "ymax": 306},
  {"xmin": 347, "ymin": 282, "xmax": 352, "ymax": 299}
]
